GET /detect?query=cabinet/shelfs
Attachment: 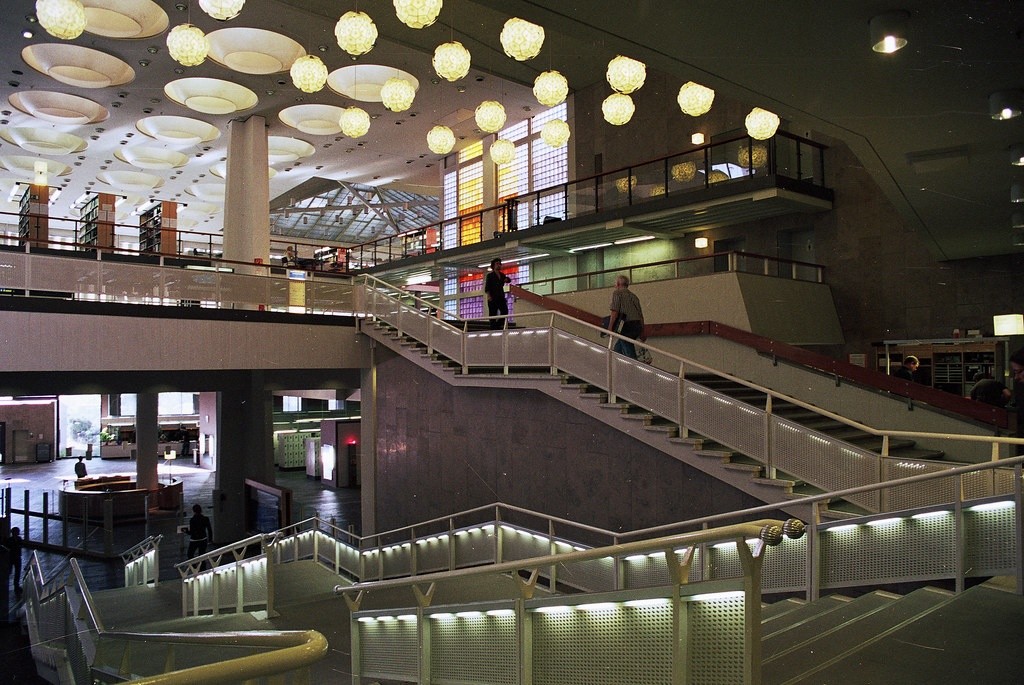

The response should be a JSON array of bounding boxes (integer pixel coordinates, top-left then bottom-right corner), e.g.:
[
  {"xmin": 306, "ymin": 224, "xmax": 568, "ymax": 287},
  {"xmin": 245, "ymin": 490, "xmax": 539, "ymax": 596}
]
[
  {"xmin": 139, "ymin": 201, "xmax": 177, "ymax": 257},
  {"xmin": 20, "ymin": 185, "xmax": 48, "ymax": 250},
  {"xmin": 79, "ymin": 193, "xmax": 115, "ymax": 254},
  {"xmin": 280, "ymin": 431, "xmax": 321, "ymax": 480},
  {"xmin": 871, "ymin": 342, "xmax": 1003, "ymax": 400}
]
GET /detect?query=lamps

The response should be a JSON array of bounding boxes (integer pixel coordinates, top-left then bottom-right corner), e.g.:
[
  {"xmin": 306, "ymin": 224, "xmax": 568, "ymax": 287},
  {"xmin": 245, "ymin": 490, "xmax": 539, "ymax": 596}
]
[
  {"xmin": 989, "ymin": 91, "xmax": 1024, "ymax": 246},
  {"xmin": 163, "ymin": 450, "xmax": 177, "ymax": 483},
  {"xmin": 0, "ymin": 0, "xmax": 781, "ymax": 203},
  {"xmin": 994, "ymin": 313, "xmax": 1024, "ymax": 336},
  {"xmin": 869, "ymin": 12, "xmax": 908, "ymax": 52}
]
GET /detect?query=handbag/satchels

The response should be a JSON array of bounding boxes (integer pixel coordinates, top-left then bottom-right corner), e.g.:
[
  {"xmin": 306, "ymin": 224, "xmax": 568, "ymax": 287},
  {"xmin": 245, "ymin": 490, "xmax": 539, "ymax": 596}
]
[{"xmin": 635, "ymin": 347, "xmax": 653, "ymax": 365}]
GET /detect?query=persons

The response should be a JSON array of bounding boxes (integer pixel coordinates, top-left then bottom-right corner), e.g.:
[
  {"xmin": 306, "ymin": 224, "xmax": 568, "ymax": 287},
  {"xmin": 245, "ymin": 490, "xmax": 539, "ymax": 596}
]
[
  {"xmin": 485, "ymin": 257, "xmax": 511, "ymax": 330},
  {"xmin": 183, "ymin": 504, "xmax": 213, "ymax": 571},
  {"xmin": 1010, "ymin": 348, "xmax": 1024, "ymax": 384},
  {"xmin": 608, "ymin": 275, "xmax": 647, "ymax": 359},
  {"xmin": 970, "ymin": 372, "xmax": 1012, "ymax": 408},
  {"xmin": 179, "ymin": 427, "xmax": 191, "ymax": 457},
  {"xmin": 286, "ymin": 246, "xmax": 299, "ymax": 266},
  {"xmin": 75, "ymin": 456, "xmax": 88, "ymax": 479},
  {"xmin": 893, "ymin": 356, "xmax": 919, "ymax": 381},
  {"xmin": 8, "ymin": 527, "xmax": 24, "ymax": 595}
]
